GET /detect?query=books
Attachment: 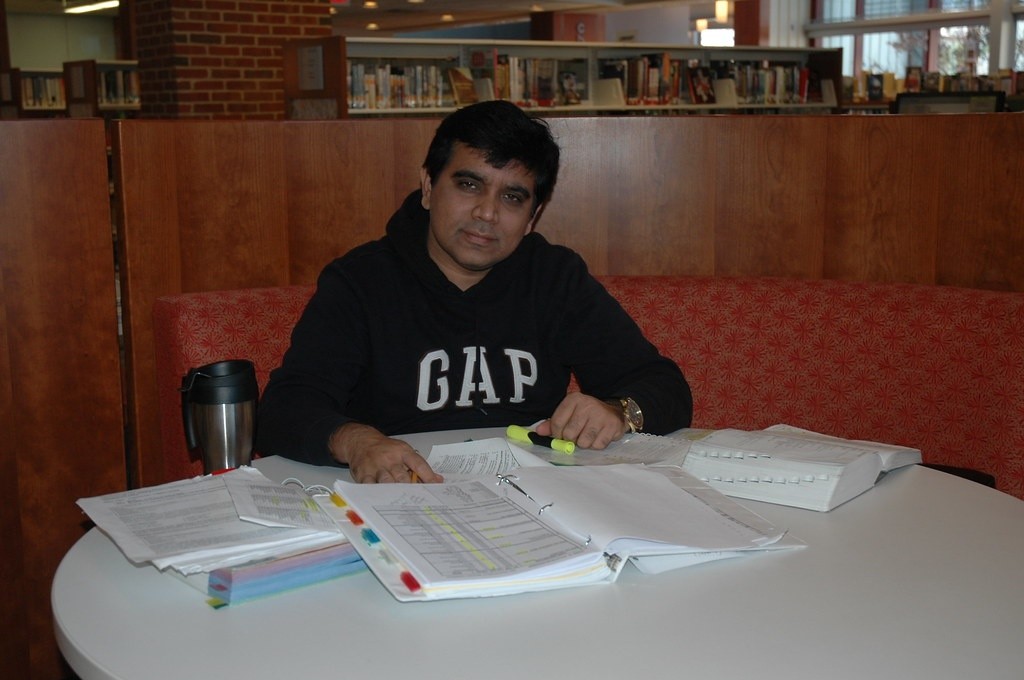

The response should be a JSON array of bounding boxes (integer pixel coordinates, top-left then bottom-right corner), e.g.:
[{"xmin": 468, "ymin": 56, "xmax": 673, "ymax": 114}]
[
  {"xmin": 312, "ymin": 462, "xmax": 807, "ymax": 604},
  {"xmin": 683, "ymin": 425, "xmax": 922, "ymax": 512},
  {"xmin": 345, "ymin": 55, "xmax": 810, "ymax": 116},
  {"xmin": 843, "ymin": 66, "xmax": 1024, "ymax": 116},
  {"xmin": 18, "ymin": 70, "xmax": 144, "ymax": 119}
]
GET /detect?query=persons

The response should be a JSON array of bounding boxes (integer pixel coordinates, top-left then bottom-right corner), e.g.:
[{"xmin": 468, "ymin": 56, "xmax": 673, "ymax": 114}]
[{"xmin": 254, "ymin": 99, "xmax": 695, "ymax": 486}]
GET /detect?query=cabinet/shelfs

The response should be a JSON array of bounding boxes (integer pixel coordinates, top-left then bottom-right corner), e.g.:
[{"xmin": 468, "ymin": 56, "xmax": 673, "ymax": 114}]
[
  {"xmin": 281, "ymin": 33, "xmax": 1023, "ymax": 119},
  {"xmin": 1, "ymin": 59, "xmax": 147, "ymax": 246}
]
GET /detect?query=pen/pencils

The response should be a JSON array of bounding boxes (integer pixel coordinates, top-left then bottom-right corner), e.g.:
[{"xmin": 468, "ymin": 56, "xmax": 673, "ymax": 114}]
[{"xmin": 411, "ymin": 449, "xmax": 419, "ymax": 483}]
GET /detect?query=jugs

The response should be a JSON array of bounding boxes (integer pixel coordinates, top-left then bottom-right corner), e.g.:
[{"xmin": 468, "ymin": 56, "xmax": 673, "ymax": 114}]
[{"xmin": 177, "ymin": 359, "xmax": 258, "ymax": 476}]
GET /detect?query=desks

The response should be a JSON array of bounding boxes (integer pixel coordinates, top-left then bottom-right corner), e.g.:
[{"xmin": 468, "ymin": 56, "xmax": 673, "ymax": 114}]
[{"xmin": 50, "ymin": 426, "xmax": 1024, "ymax": 680}]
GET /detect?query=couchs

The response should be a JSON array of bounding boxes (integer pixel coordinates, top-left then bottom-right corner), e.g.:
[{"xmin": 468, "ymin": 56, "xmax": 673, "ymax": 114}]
[{"xmin": 152, "ymin": 279, "xmax": 1023, "ymax": 503}]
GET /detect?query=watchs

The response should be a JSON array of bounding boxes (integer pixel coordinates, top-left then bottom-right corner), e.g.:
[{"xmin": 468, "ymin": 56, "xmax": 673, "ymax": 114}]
[{"xmin": 607, "ymin": 395, "xmax": 644, "ymax": 434}]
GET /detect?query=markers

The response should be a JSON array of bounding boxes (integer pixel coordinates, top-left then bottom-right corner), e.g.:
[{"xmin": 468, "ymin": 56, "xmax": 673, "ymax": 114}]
[{"xmin": 506, "ymin": 424, "xmax": 576, "ymax": 455}]
